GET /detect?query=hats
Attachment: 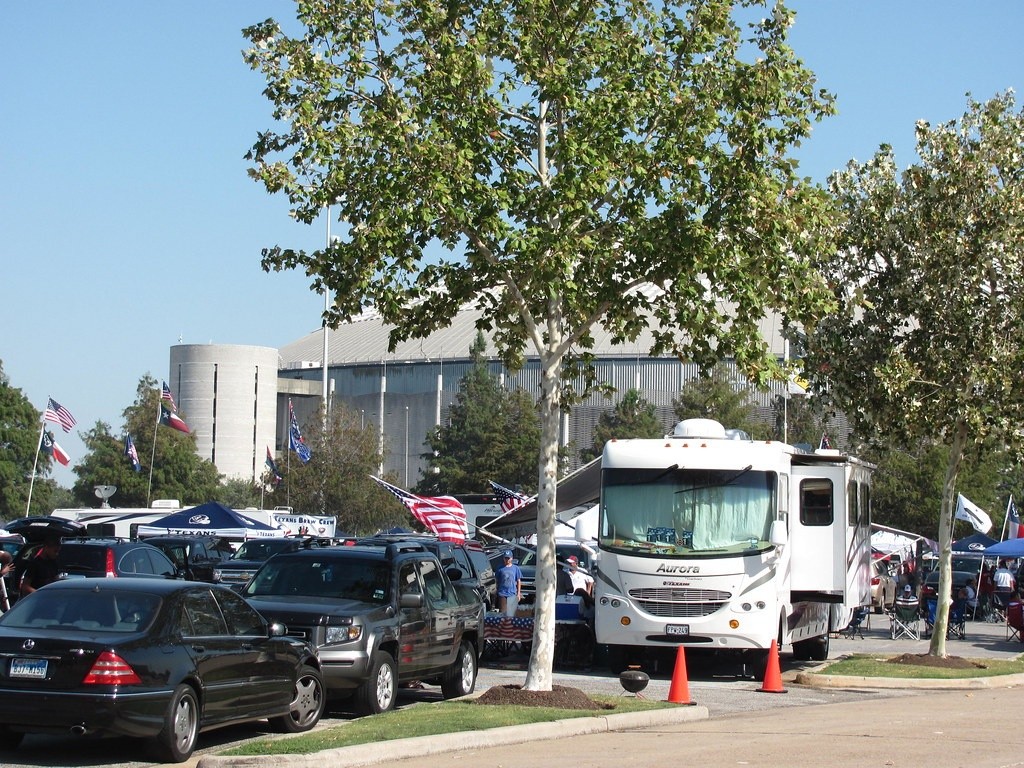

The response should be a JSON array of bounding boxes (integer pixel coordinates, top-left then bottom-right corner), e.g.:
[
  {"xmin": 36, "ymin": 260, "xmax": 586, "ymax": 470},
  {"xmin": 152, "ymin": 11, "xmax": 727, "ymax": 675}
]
[
  {"xmin": 503, "ymin": 549, "xmax": 513, "ymax": 558},
  {"xmin": 904, "ymin": 584, "xmax": 912, "ymax": 591},
  {"xmin": 564, "ymin": 555, "xmax": 579, "ymax": 563}
]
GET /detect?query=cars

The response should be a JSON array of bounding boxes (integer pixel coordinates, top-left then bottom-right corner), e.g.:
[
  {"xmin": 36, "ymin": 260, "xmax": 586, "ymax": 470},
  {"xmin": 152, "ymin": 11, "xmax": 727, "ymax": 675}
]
[
  {"xmin": 868, "ymin": 542, "xmax": 1024, "ymax": 621},
  {"xmin": 0, "ymin": 576, "xmax": 329, "ymax": 764}
]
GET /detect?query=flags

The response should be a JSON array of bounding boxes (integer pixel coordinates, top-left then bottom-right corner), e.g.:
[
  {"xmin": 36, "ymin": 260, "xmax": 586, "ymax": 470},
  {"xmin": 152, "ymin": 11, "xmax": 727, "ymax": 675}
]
[
  {"xmin": 156, "ymin": 380, "xmax": 191, "ymax": 433},
  {"xmin": 954, "ymin": 491, "xmax": 993, "ymax": 534},
  {"xmin": 39, "ymin": 429, "xmax": 71, "ymax": 467},
  {"xmin": 373, "ymin": 479, "xmax": 470, "ymax": 546},
  {"xmin": 124, "ymin": 430, "xmax": 141, "ymax": 471},
  {"xmin": 491, "ymin": 483, "xmax": 536, "ymax": 542},
  {"xmin": 289, "ymin": 400, "xmax": 312, "ymax": 463},
  {"xmin": 44, "ymin": 396, "xmax": 78, "ymax": 434},
  {"xmin": 265, "ymin": 445, "xmax": 283, "ymax": 480},
  {"xmin": 1008, "ymin": 500, "xmax": 1023, "ymax": 538}
]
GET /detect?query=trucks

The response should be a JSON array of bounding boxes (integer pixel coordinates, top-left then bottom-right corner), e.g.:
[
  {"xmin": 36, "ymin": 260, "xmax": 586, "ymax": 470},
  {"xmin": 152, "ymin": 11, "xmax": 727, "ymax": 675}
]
[{"xmin": 478, "ymin": 417, "xmax": 878, "ymax": 682}]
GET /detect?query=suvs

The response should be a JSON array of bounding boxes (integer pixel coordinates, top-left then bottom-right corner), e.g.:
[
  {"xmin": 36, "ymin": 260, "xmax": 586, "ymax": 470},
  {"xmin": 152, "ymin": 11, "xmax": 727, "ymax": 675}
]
[
  {"xmin": 229, "ymin": 538, "xmax": 487, "ymax": 715},
  {"xmin": 1, "ymin": 515, "xmax": 597, "ymax": 655}
]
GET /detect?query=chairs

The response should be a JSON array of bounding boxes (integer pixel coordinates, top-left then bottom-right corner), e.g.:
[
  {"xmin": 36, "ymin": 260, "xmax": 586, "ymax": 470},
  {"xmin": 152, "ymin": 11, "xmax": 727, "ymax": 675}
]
[
  {"xmin": 294, "ymin": 564, "xmax": 387, "ymax": 600},
  {"xmin": 890, "ymin": 594, "xmax": 1024, "ymax": 646},
  {"xmin": 34, "ymin": 617, "xmax": 140, "ymax": 633},
  {"xmin": 835, "ymin": 600, "xmax": 873, "ymax": 640}
]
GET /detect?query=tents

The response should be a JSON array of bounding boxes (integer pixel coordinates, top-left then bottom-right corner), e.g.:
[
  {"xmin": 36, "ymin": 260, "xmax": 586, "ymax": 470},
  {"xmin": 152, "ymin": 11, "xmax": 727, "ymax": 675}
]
[
  {"xmin": 870, "ymin": 530, "xmax": 1024, "ymax": 580},
  {"xmin": 136, "ymin": 502, "xmax": 286, "ymax": 544}
]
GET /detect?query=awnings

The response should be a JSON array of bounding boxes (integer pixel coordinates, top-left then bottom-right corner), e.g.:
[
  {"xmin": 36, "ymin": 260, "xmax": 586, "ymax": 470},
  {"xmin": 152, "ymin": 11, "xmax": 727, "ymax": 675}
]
[
  {"xmin": 481, "ymin": 456, "xmax": 601, "ymax": 546},
  {"xmin": 871, "ymin": 524, "xmax": 938, "ymax": 580}
]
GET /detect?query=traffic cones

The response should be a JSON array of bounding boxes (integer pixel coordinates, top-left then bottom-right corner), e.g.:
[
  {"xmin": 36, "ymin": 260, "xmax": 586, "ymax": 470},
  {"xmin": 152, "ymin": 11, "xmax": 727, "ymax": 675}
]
[
  {"xmin": 660, "ymin": 643, "xmax": 698, "ymax": 705},
  {"xmin": 754, "ymin": 638, "xmax": 789, "ymax": 694}
]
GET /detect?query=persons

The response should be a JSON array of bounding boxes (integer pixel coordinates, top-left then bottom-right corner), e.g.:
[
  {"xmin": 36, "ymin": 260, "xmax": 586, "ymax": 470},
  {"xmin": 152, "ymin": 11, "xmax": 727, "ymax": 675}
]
[
  {"xmin": 0, "ymin": 534, "xmax": 63, "ymax": 616},
  {"xmin": 556, "ymin": 555, "xmax": 594, "ymax": 624},
  {"xmin": 954, "ymin": 559, "xmax": 1024, "ymax": 644},
  {"xmin": 890, "ymin": 561, "xmax": 969, "ymax": 634},
  {"xmin": 496, "ymin": 550, "xmax": 523, "ymax": 615},
  {"xmin": 239, "ymin": 639, "xmax": 282, "ymax": 717}
]
